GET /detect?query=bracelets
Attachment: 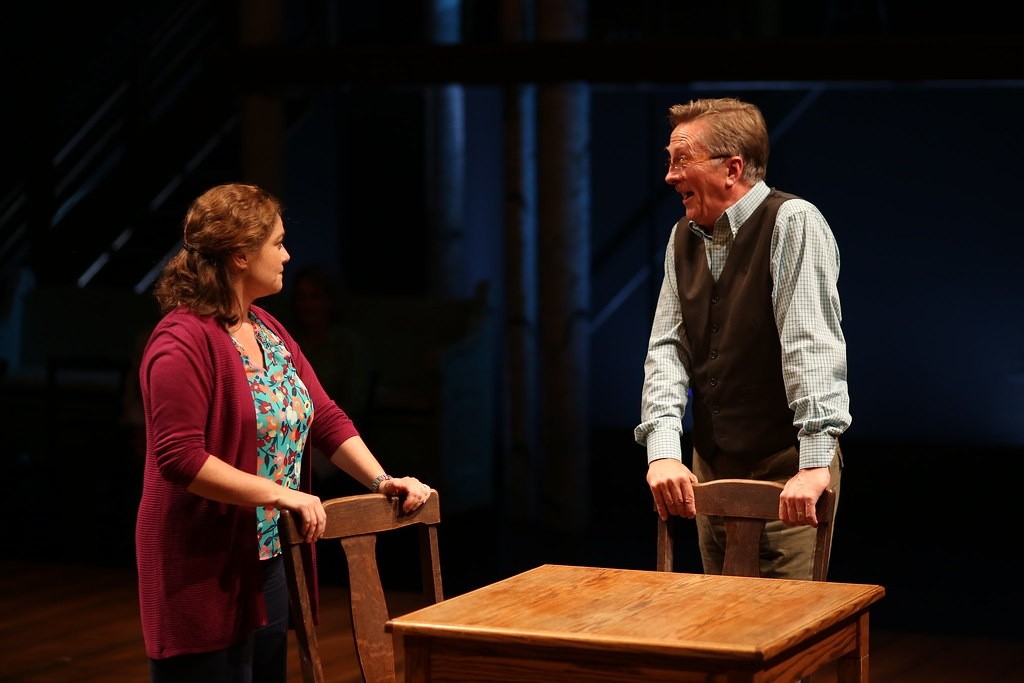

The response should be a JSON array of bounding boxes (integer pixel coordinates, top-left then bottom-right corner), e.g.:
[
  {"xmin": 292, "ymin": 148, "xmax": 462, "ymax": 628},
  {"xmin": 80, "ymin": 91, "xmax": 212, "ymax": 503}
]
[{"xmin": 372, "ymin": 474, "xmax": 393, "ymax": 493}]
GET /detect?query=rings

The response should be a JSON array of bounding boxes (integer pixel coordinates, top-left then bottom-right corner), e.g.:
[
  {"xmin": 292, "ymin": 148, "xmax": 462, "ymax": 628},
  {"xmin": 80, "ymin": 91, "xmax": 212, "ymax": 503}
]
[{"xmin": 796, "ymin": 512, "xmax": 803, "ymax": 516}]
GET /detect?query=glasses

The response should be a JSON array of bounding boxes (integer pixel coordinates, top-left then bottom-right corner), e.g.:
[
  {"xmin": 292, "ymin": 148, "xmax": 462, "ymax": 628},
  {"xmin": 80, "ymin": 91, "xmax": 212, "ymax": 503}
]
[{"xmin": 664, "ymin": 153, "xmax": 732, "ymax": 171}]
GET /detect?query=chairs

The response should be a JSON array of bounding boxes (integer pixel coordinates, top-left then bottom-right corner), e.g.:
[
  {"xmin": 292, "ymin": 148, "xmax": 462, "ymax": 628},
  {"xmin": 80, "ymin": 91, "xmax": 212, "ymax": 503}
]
[
  {"xmin": 657, "ymin": 479, "xmax": 835, "ymax": 581},
  {"xmin": 277, "ymin": 488, "xmax": 445, "ymax": 683}
]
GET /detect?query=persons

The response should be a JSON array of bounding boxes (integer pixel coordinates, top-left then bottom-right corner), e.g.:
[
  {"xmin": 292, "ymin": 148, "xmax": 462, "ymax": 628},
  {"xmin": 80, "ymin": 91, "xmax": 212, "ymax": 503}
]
[
  {"xmin": 633, "ymin": 97, "xmax": 852, "ymax": 580},
  {"xmin": 135, "ymin": 182, "xmax": 432, "ymax": 683}
]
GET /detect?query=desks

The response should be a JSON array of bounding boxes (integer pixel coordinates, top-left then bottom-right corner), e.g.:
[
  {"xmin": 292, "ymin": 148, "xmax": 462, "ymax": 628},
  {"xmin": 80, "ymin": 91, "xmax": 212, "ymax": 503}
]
[{"xmin": 383, "ymin": 563, "xmax": 886, "ymax": 683}]
